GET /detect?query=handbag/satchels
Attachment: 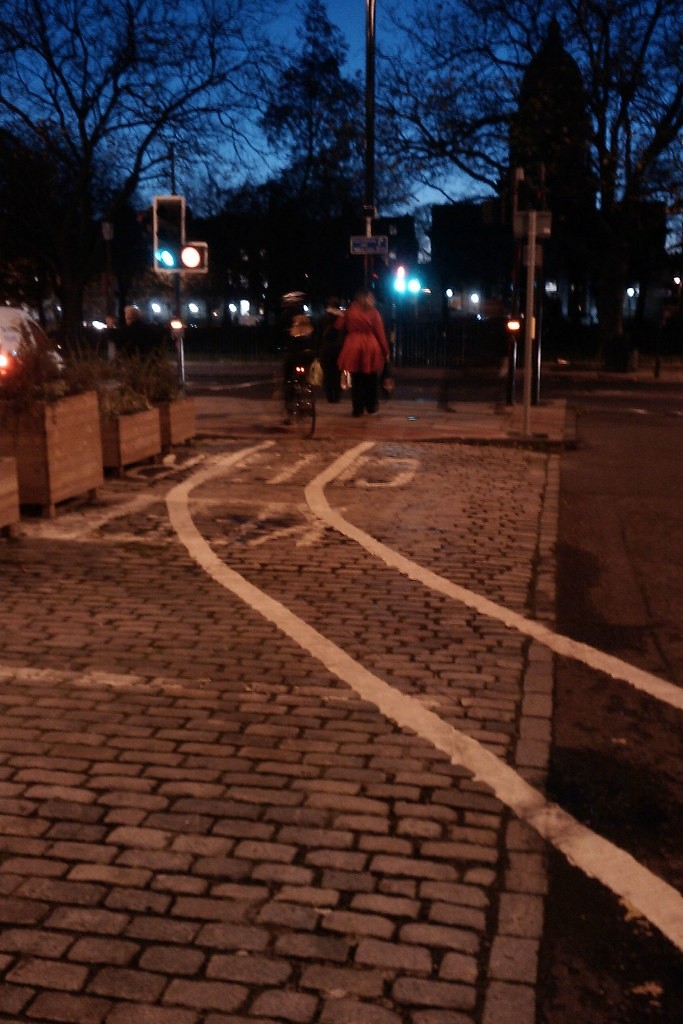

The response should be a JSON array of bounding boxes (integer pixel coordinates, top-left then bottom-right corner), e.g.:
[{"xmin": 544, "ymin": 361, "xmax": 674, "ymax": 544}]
[
  {"xmin": 307, "ymin": 362, "xmax": 324, "ymax": 387},
  {"xmin": 341, "ymin": 371, "xmax": 352, "ymax": 391}
]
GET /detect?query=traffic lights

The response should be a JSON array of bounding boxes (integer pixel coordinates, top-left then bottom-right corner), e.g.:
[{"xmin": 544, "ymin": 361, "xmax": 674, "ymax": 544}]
[
  {"xmin": 152, "ymin": 195, "xmax": 210, "ymax": 275},
  {"xmin": 504, "ymin": 317, "xmax": 521, "ymax": 334},
  {"xmin": 391, "ymin": 264, "xmax": 422, "ymax": 296},
  {"xmin": 168, "ymin": 315, "xmax": 183, "ymax": 333}
]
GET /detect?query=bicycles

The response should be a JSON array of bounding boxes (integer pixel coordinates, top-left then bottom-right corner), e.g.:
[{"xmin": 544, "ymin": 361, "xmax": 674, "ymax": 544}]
[{"xmin": 275, "ymin": 345, "xmax": 316, "ymax": 440}]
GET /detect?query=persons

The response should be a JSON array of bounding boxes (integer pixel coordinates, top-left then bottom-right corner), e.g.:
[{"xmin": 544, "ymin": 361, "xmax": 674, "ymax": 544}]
[
  {"xmin": 105, "ymin": 304, "xmax": 157, "ymax": 362},
  {"xmin": 314, "ymin": 289, "xmax": 479, "ymax": 419}
]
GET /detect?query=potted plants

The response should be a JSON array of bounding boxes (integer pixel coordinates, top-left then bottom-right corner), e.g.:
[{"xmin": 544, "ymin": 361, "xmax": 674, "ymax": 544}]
[{"xmin": 0, "ymin": 352, "xmax": 196, "ymax": 529}]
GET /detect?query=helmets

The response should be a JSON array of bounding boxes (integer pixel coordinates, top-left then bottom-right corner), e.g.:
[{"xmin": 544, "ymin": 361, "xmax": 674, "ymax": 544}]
[{"xmin": 282, "ymin": 291, "xmax": 307, "ymax": 306}]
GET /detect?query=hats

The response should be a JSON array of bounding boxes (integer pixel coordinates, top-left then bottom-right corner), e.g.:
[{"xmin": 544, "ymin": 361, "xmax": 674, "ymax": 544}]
[
  {"xmin": 290, "ymin": 315, "xmax": 313, "ymax": 337},
  {"xmin": 328, "ymin": 298, "xmax": 342, "ymax": 308}
]
